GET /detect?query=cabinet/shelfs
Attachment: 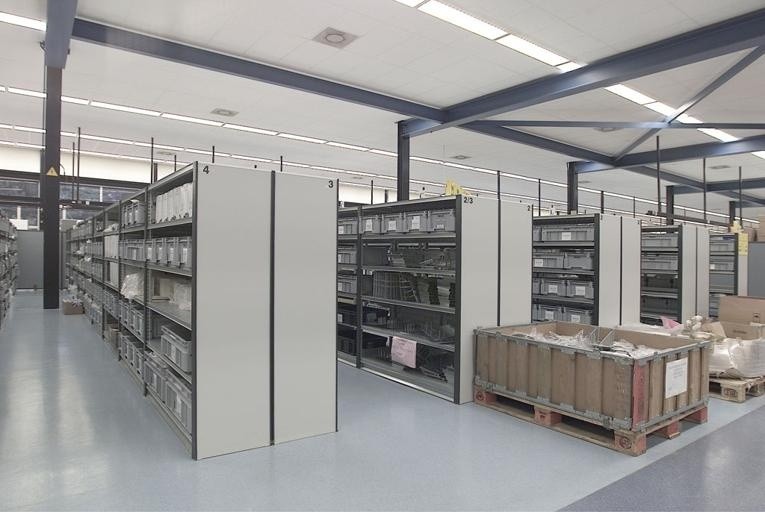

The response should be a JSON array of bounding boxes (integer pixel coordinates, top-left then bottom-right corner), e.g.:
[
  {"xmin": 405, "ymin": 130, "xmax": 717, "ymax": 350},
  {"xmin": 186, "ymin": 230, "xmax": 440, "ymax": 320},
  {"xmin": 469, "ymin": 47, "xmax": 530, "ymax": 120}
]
[
  {"xmin": 697, "ymin": 226, "xmax": 709, "ymax": 317},
  {"xmin": 532, "ymin": 213, "xmax": 621, "ymax": 327},
  {"xmin": 144, "ymin": 161, "xmax": 273, "ymax": 461},
  {"xmin": 500, "ymin": 197, "xmax": 533, "ymax": 326},
  {"xmin": 273, "ymin": 169, "xmax": 339, "ymax": 444},
  {"xmin": 65, "ymin": 202, "xmax": 119, "ymax": 361},
  {"xmin": 119, "ymin": 189, "xmax": 143, "ymax": 391},
  {"xmin": 0, "ymin": 204, "xmax": 21, "ymax": 327},
  {"xmin": 621, "ymin": 216, "xmax": 641, "ymax": 326},
  {"xmin": 640, "ymin": 222, "xmax": 697, "ymax": 326},
  {"xmin": 337, "ymin": 205, "xmax": 359, "ymax": 368},
  {"xmin": 359, "ymin": 195, "xmax": 498, "ymax": 405},
  {"xmin": 710, "ymin": 233, "xmax": 748, "ymax": 317}
]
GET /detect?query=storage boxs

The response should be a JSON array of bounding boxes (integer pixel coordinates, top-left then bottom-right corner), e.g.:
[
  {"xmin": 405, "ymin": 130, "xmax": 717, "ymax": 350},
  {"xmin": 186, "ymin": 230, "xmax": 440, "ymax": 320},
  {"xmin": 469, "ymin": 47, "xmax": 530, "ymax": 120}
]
[
  {"xmin": 473, "ymin": 319, "xmax": 711, "ymax": 457},
  {"xmin": 719, "ymin": 296, "xmax": 765, "ymax": 340}
]
